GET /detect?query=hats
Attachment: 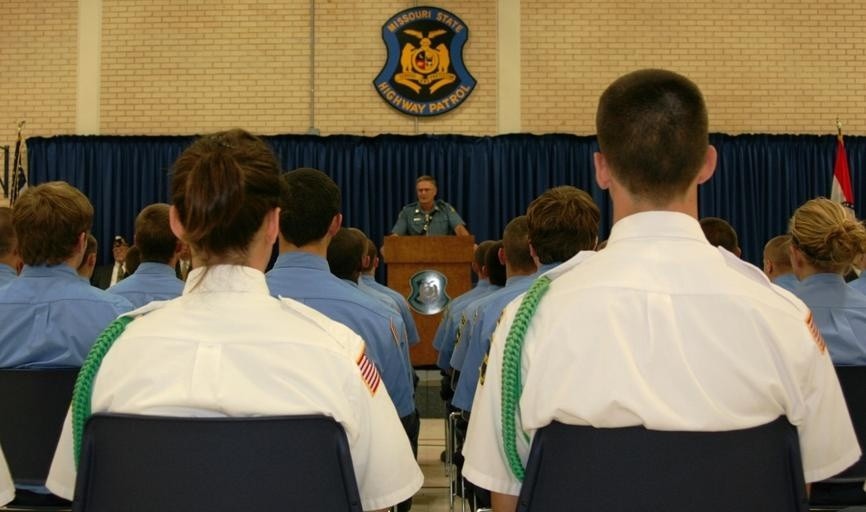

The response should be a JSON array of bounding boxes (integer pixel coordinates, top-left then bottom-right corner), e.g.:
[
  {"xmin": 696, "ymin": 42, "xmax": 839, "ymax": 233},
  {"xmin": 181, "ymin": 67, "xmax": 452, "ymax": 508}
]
[{"xmin": 113, "ymin": 236, "xmax": 129, "ymax": 246}]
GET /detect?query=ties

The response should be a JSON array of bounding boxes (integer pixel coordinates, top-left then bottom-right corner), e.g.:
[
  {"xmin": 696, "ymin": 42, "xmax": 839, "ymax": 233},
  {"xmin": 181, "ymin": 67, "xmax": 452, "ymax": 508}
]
[{"xmin": 117, "ymin": 263, "xmax": 124, "ymax": 282}]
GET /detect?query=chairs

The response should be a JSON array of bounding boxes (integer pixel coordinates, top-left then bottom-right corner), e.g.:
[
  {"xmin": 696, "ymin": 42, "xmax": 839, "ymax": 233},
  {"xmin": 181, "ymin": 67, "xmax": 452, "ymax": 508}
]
[
  {"xmin": 72, "ymin": 411, "xmax": 365, "ymax": 512},
  {"xmin": 440, "ymin": 362, "xmax": 491, "ymax": 510},
  {"xmin": 0, "ymin": 364, "xmax": 84, "ymax": 511},
  {"xmin": 514, "ymin": 415, "xmax": 809, "ymax": 512},
  {"xmin": 809, "ymin": 364, "xmax": 865, "ymax": 511}
]
